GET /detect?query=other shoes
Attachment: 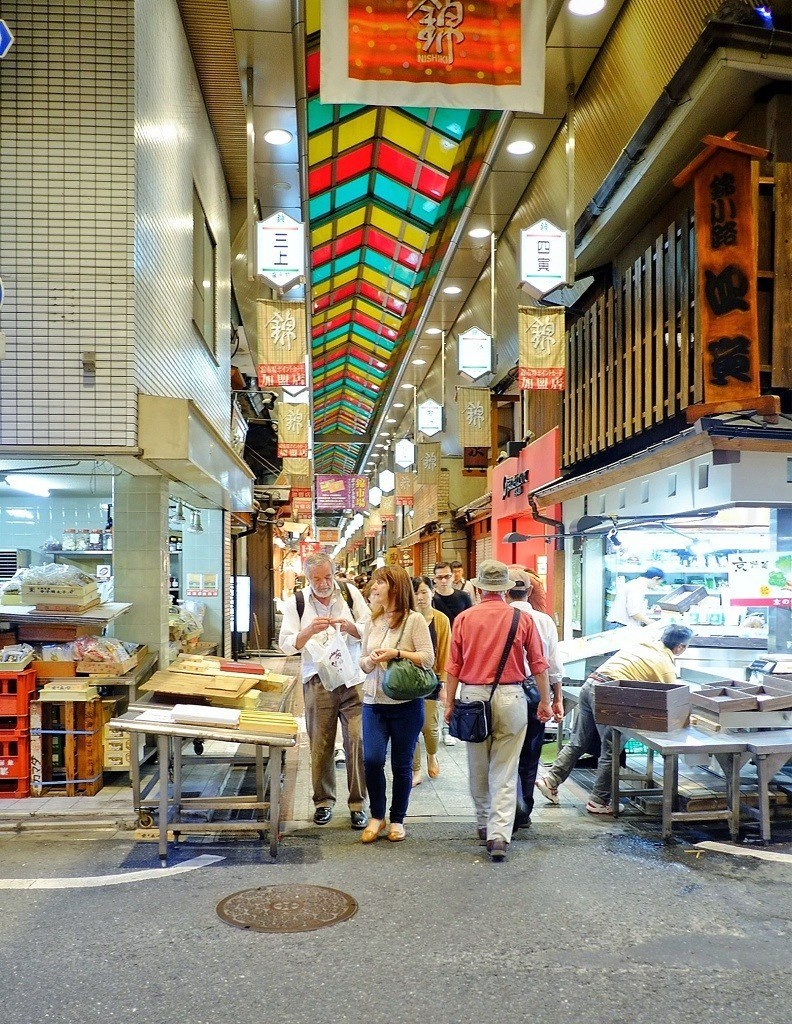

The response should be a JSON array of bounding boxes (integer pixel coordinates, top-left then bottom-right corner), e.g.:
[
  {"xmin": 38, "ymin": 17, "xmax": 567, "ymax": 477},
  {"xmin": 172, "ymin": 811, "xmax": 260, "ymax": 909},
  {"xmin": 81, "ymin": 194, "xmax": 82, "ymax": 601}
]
[
  {"xmin": 387, "ymin": 823, "xmax": 405, "ymax": 841},
  {"xmin": 478, "ymin": 839, "xmax": 509, "ymax": 857},
  {"xmin": 362, "ymin": 818, "xmax": 386, "ymax": 842},
  {"xmin": 426, "ymin": 754, "xmax": 440, "ymax": 778},
  {"xmin": 519, "ymin": 816, "xmax": 532, "ymax": 828},
  {"xmin": 443, "ymin": 734, "xmax": 456, "ymax": 746},
  {"xmin": 478, "ymin": 827, "xmax": 487, "ymax": 840},
  {"xmin": 411, "ymin": 776, "xmax": 422, "ymax": 788}
]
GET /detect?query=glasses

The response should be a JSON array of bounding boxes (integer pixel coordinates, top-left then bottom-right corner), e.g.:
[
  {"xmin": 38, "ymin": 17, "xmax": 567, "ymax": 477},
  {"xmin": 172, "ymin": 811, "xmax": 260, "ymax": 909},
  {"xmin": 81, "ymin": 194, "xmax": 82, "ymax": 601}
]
[{"xmin": 436, "ymin": 574, "xmax": 452, "ymax": 580}]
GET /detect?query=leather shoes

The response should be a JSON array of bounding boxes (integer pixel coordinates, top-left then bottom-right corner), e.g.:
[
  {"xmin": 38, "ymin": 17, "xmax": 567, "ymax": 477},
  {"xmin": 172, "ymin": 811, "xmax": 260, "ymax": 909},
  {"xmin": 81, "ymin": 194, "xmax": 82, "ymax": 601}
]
[
  {"xmin": 350, "ymin": 811, "xmax": 367, "ymax": 828},
  {"xmin": 313, "ymin": 806, "xmax": 332, "ymax": 824}
]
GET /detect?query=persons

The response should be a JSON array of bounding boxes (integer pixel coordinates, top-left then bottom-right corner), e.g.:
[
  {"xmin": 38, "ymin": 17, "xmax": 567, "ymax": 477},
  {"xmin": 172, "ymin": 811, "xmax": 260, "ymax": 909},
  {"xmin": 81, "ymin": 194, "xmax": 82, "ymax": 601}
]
[
  {"xmin": 450, "ymin": 561, "xmax": 475, "ymax": 606},
  {"xmin": 359, "ymin": 565, "xmax": 435, "ymax": 843},
  {"xmin": 411, "ymin": 576, "xmax": 451, "ymax": 788},
  {"xmin": 534, "ymin": 620, "xmax": 692, "ymax": 814},
  {"xmin": 432, "ymin": 561, "xmax": 472, "ymax": 746},
  {"xmin": 504, "ymin": 570, "xmax": 565, "ymax": 835},
  {"xmin": 333, "ymin": 571, "xmax": 379, "ymax": 763},
  {"xmin": 507, "ymin": 565, "xmax": 546, "ymax": 613},
  {"xmin": 279, "ymin": 552, "xmax": 373, "ymax": 827},
  {"xmin": 443, "ymin": 560, "xmax": 553, "ymax": 859},
  {"xmin": 602, "ymin": 567, "xmax": 664, "ymax": 655}
]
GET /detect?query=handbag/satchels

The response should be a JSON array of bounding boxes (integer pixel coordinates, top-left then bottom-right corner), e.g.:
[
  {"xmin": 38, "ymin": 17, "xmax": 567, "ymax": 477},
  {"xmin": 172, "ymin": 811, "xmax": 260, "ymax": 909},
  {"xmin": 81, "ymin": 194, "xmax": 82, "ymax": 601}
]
[
  {"xmin": 304, "ymin": 622, "xmax": 355, "ymax": 692},
  {"xmin": 521, "ymin": 674, "xmax": 549, "ymax": 704},
  {"xmin": 448, "ymin": 699, "xmax": 494, "ymax": 743},
  {"xmin": 382, "ymin": 656, "xmax": 438, "ymax": 700}
]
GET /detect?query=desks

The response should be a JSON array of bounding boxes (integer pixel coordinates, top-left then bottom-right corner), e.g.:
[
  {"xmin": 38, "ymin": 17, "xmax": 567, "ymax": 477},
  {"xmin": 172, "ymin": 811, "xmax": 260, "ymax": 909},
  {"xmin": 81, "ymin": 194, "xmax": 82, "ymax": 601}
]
[
  {"xmin": 732, "ymin": 730, "xmax": 792, "ymax": 841},
  {"xmin": 556, "ymin": 687, "xmax": 748, "ymax": 841}
]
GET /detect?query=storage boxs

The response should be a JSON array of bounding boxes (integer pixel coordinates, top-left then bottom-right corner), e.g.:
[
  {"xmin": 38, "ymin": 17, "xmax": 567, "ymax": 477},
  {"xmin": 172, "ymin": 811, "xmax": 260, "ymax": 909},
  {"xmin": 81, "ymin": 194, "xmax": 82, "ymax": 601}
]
[
  {"xmin": 594, "ymin": 680, "xmax": 690, "ymax": 732},
  {"xmin": 692, "ymin": 680, "xmax": 792, "ymax": 714},
  {"xmin": 0, "ymin": 621, "xmax": 157, "ymax": 798},
  {"xmin": 658, "ymin": 585, "xmax": 708, "ymax": 611}
]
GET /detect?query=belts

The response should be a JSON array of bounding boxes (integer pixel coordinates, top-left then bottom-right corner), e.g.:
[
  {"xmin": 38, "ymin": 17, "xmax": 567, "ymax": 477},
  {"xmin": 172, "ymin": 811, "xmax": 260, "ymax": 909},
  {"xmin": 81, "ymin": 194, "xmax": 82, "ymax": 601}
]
[{"xmin": 592, "ymin": 671, "xmax": 613, "ymax": 682}]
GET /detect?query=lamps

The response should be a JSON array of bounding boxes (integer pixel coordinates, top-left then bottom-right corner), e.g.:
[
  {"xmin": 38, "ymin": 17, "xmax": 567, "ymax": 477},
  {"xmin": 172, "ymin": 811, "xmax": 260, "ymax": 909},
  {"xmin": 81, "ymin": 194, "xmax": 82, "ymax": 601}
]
[
  {"xmin": 172, "ymin": 502, "xmax": 185, "ymax": 524},
  {"xmin": 569, "ymin": 511, "xmax": 717, "ymax": 532},
  {"xmin": 0, "ymin": 472, "xmax": 50, "ymax": 497},
  {"xmin": 607, "ymin": 525, "xmax": 623, "ymax": 548},
  {"xmin": 257, "ymin": 509, "xmax": 285, "ymax": 528},
  {"xmin": 187, "ymin": 511, "xmax": 203, "ymax": 534},
  {"xmin": 502, "ymin": 533, "xmax": 608, "ymax": 556},
  {"xmin": 382, "ymin": 541, "xmax": 402, "ymax": 557},
  {"xmin": 571, "ymin": 536, "xmax": 583, "ymax": 555},
  {"xmin": 233, "ymin": 390, "xmax": 280, "ymax": 431},
  {"xmin": 495, "ymin": 430, "xmax": 536, "ymax": 464},
  {"xmin": 465, "ymin": 507, "xmax": 491, "ymax": 522}
]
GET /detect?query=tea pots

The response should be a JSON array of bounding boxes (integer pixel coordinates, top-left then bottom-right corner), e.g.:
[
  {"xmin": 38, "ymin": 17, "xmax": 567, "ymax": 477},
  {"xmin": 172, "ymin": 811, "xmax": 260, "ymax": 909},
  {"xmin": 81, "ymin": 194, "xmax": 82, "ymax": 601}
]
[{"xmin": 44, "ymin": 536, "xmax": 60, "ymax": 550}]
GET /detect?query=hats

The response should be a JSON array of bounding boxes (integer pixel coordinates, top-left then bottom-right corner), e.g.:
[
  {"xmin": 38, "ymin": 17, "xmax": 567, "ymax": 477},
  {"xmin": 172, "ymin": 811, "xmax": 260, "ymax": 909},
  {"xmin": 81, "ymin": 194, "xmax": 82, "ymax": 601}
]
[
  {"xmin": 507, "ymin": 570, "xmax": 531, "ymax": 590},
  {"xmin": 470, "ymin": 559, "xmax": 516, "ymax": 591}
]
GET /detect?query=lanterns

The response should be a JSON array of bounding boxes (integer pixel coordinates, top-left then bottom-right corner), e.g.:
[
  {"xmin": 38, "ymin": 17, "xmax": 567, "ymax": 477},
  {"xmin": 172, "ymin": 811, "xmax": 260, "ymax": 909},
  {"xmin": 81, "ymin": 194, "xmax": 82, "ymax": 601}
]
[{"xmin": 285, "ymin": 570, "xmax": 295, "ymax": 590}]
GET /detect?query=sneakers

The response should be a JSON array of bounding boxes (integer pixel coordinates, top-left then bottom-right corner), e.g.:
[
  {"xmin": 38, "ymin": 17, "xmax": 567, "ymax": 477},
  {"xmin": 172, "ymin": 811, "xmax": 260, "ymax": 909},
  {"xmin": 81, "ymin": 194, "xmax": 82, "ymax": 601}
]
[
  {"xmin": 586, "ymin": 798, "xmax": 624, "ymax": 814},
  {"xmin": 534, "ymin": 775, "xmax": 560, "ymax": 804}
]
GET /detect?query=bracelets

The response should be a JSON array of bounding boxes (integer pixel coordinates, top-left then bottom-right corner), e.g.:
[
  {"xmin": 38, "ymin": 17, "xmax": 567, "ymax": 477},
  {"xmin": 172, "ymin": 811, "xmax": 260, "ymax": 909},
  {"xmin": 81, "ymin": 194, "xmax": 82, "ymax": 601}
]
[
  {"xmin": 397, "ymin": 650, "xmax": 400, "ymax": 657},
  {"xmin": 365, "ymin": 657, "xmax": 374, "ymax": 669}
]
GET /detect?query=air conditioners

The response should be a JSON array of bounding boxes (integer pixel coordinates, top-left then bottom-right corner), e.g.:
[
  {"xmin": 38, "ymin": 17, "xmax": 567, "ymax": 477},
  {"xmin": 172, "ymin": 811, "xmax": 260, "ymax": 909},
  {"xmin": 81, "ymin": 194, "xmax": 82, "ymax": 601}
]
[{"xmin": 0, "ymin": 548, "xmax": 27, "ymax": 583}]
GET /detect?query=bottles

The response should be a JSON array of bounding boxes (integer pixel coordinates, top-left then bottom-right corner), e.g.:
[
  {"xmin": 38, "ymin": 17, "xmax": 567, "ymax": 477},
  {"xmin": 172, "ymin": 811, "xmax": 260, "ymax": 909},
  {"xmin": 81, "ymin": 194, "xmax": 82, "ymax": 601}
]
[
  {"xmin": 61, "ymin": 529, "xmax": 113, "ymax": 551},
  {"xmin": 170, "ymin": 577, "xmax": 178, "ymax": 588}
]
